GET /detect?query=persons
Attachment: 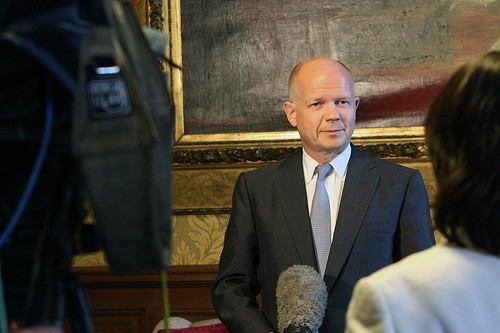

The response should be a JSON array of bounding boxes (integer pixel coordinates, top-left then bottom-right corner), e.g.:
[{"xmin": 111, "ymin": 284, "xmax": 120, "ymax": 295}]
[
  {"xmin": 211, "ymin": 58, "xmax": 435, "ymax": 333},
  {"xmin": 344, "ymin": 41, "xmax": 500, "ymax": 333}
]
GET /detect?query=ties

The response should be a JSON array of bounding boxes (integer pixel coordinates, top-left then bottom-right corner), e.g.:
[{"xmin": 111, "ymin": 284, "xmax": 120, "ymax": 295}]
[{"xmin": 310, "ymin": 164, "xmax": 332, "ymax": 280}]
